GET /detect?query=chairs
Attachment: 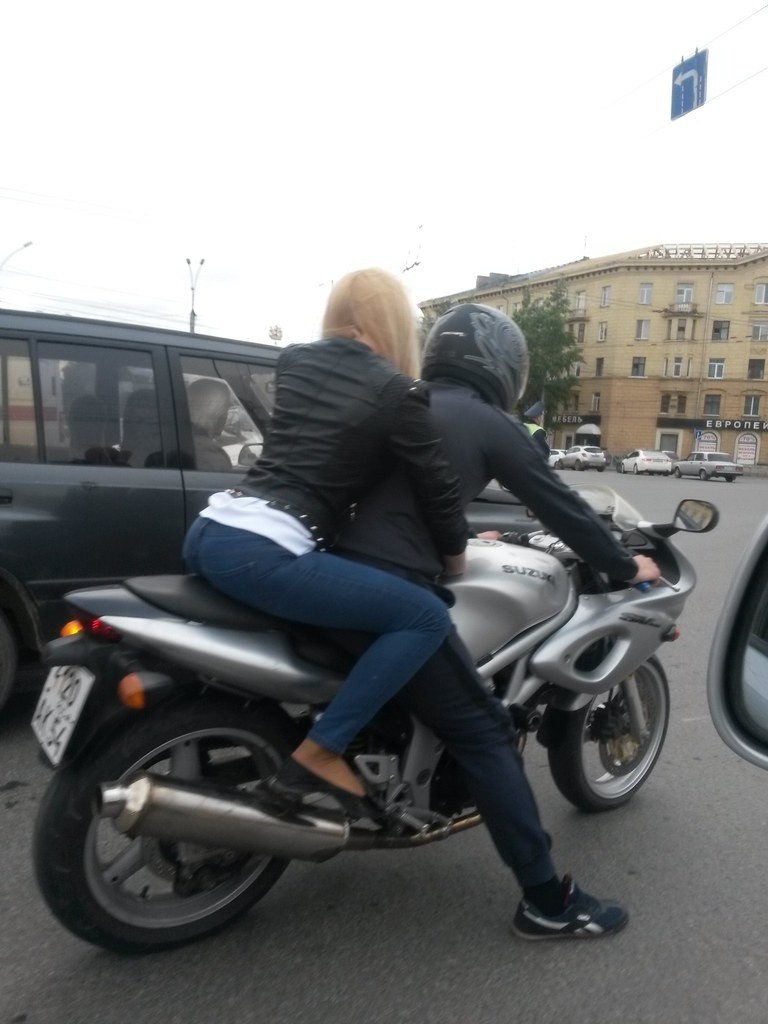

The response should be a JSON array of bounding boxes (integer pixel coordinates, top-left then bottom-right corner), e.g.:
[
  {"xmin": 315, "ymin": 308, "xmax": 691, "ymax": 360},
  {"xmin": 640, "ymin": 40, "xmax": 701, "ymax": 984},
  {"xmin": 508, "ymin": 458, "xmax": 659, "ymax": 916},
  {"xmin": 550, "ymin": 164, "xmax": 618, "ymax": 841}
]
[{"xmin": 68, "ymin": 378, "xmax": 235, "ymax": 470}]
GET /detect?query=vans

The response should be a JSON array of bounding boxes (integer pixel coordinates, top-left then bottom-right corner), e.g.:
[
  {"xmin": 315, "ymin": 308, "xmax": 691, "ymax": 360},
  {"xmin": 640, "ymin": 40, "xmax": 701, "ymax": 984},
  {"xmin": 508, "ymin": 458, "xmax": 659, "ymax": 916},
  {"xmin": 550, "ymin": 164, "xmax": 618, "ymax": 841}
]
[{"xmin": 0, "ymin": 308, "xmax": 283, "ymax": 715}]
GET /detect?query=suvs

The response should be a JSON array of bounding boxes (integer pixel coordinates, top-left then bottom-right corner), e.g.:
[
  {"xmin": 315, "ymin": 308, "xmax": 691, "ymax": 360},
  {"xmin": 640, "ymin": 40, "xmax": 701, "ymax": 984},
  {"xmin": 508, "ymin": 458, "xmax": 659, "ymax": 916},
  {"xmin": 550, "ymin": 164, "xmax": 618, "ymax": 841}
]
[{"xmin": 558, "ymin": 444, "xmax": 607, "ymax": 473}]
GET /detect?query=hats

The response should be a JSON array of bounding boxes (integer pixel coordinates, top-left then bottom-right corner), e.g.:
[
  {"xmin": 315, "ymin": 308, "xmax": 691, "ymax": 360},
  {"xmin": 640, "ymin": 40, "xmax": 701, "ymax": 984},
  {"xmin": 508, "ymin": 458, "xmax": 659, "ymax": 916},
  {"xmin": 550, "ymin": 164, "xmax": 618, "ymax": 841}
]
[{"xmin": 524, "ymin": 401, "xmax": 545, "ymax": 418}]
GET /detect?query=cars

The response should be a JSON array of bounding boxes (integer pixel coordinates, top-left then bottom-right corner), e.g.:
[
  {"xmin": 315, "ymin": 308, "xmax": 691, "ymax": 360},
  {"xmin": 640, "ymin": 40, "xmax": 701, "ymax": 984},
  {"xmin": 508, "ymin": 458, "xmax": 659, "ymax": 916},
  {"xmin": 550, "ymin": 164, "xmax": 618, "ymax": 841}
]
[
  {"xmin": 619, "ymin": 448, "xmax": 674, "ymax": 477},
  {"xmin": 673, "ymin": 451, "xmax": 744, "ymax": 483},
  {"xmin": 549, "ymin": 448, "xmax": 566, "ymax": 469}
]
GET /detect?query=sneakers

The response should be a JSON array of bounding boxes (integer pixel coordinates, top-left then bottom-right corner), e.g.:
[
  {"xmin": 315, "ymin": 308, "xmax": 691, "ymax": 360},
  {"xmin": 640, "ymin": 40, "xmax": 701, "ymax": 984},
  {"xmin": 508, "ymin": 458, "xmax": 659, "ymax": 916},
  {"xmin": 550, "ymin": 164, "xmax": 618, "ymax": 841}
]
[{"xmin": 511, "ymin": 874, "xmax": 630, "ymax": 940}]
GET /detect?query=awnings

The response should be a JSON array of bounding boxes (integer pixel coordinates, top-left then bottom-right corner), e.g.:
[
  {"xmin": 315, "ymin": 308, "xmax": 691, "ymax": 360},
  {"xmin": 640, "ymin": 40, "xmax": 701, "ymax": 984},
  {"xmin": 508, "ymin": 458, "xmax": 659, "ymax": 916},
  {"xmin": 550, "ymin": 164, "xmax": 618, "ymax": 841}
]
[{"xmin": 576, "ymin": 424, "xmax": 601, "ymax": 435}]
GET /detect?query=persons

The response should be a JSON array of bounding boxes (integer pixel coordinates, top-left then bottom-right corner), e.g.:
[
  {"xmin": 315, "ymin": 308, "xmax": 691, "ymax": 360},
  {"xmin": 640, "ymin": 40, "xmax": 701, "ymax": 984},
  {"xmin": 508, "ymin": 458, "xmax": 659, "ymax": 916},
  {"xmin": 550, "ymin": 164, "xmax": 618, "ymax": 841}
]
[
  {"xmin": 186, "ymin": 377, "xmax": 235, "ymax": 471},
  {"xmin": 119, "ymin": 389, "xmax": 164, "ymax": 469},
  {"xmin": 322, "ymin": 302, "xmax": 659, "ymax": 941},
  {"xmin": 178, "ymin": 266, "xmax": 477, "ymax": 816},
  {"xmin": 521, "ymin": 401, "xmax": 550, "ymax": 462}
]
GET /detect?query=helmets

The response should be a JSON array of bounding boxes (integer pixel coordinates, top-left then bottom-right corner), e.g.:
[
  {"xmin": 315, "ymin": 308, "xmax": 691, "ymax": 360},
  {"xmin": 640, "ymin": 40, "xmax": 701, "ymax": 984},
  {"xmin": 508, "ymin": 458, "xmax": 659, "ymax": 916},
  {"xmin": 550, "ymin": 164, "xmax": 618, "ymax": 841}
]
[{"xmin": 420, "ymin": 303, "xmax": 530, "ymax": 414}]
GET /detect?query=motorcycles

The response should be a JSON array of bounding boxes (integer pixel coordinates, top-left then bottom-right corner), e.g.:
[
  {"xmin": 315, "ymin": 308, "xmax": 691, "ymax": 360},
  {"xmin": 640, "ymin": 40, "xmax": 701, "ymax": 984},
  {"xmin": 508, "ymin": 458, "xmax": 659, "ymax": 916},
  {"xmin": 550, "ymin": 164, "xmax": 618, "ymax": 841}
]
[{"xmin": 29, "ymin": 482, "xmax": 719, "ymax": 961}]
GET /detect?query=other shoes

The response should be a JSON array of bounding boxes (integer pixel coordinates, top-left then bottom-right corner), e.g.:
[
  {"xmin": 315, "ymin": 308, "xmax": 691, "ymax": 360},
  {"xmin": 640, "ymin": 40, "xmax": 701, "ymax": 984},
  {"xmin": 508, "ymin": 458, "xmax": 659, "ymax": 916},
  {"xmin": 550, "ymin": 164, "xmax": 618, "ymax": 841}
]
[{"xmin": 281, "ymin": 737, "xmax": 372, "ymax": 802}]
